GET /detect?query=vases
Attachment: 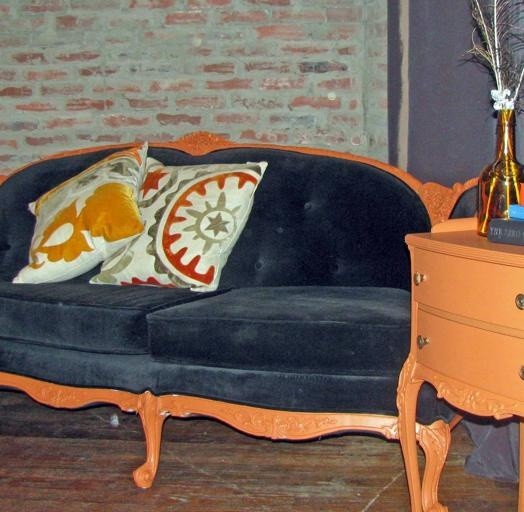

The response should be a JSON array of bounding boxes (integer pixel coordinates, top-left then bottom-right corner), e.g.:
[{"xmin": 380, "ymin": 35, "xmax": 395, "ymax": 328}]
[{"xmin": 477, "ymin": 111, "xmax": 523, "ymax": 234}]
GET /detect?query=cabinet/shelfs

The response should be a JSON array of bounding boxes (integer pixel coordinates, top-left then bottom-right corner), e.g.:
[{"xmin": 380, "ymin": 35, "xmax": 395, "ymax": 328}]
[{"xmin": 394, "ymin": 214, "xmax": 523, "ymax": 510}]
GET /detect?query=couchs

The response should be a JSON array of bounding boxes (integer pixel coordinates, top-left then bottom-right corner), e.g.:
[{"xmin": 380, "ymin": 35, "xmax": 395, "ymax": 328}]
[{"xmin": 2, "ymin": 137, "xmax": 484, "ymax": 512}]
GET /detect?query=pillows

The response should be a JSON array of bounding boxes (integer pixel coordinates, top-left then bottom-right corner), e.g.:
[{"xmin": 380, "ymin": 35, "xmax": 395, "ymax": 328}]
[
  {"xmin": 89, "ymin": 152, "xmax": 269, "ymax": 292},
  {"xmin": 11, "ymin": 139, "xmax": 147, "ymax": 285}
]
